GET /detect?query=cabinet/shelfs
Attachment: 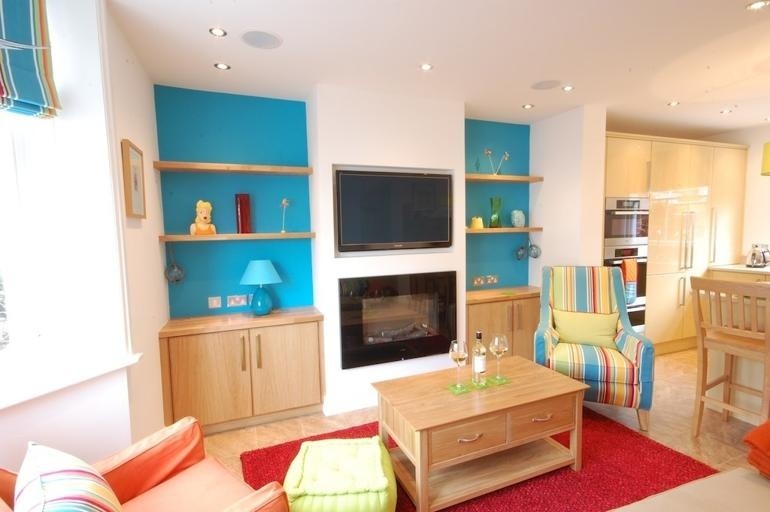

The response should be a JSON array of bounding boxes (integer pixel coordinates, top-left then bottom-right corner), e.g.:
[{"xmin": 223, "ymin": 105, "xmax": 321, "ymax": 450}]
[
  {"xmin": 607, "ymin": 130, "xmax": 650, "ymax": 196},
  {"xmin": 641, "ymin": 135, "xmax": 715, "ymax": 274},
  {"xmin": 645, "ymin": 266, "xmax": 704, "ymax": 357},
  {"xmin": 159, "ymin": 305, "xmax": 324, "ymax": 439},
  {"xmin": 715, "ymin": 140, "xmax": 749, "ymax": 265},
  {"xmin": 151, "ymin": 159, "xmax": 318, "ymax": 243},
  {"xmin": 466, "ymin": 286, "xmax": 541, "ymax": 362},
  {"xmin": 465, "ymin": 171, "xmax": 545, "ymax": 235}
]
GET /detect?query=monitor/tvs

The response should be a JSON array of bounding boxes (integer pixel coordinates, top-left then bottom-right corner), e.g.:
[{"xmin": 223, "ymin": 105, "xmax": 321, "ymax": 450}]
[{"xmin": 335, "ymin": 170, "xmax": 452, "ymax": 253}]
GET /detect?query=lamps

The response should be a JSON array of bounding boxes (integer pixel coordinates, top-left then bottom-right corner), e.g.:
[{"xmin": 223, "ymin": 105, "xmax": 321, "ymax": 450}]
[{"xmin": 238, "ymin": 258, "xmax": 283, "ymax": 316}]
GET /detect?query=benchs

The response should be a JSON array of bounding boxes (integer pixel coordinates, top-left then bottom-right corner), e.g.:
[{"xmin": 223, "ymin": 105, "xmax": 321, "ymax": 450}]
[{"xmin": 607, "ymin": 463, "xmax": 770, "ymax": 510}]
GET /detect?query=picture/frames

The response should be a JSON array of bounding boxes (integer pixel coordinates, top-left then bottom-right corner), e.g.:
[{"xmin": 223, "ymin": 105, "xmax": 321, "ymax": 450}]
[{"xmin": 120, "ymin": 138, "xmax": 147, "ymax": 220}]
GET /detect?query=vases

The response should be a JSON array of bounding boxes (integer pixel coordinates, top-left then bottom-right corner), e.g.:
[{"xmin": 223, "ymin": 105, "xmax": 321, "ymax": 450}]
[{"xmin": 488, "ymin": 196, "xmax": 503, "ymax": 228}]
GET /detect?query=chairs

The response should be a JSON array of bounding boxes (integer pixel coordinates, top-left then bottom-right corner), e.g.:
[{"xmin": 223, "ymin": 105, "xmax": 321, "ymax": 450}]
[
  {"xmin": 534, "ymin": 262, "xmax": 656, "ymax": 434},
  {"xmin": 0, "ymin": 411, "xmax": 293, "ymax": 512},
  {"xmin": 689, "ymin": 273, "xmax": 769, "ymax": 454}
]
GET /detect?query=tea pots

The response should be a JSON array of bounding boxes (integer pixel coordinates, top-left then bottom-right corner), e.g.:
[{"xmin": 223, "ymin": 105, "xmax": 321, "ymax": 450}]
[{"xmin": 745, "ymin": 245, "xmax": 769, "ymax": 268}]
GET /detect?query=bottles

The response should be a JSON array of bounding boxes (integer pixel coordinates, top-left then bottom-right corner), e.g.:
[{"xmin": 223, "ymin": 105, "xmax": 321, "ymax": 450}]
[
  {"xmin": 510, "ymin": 209, "xmax": 526, "ymax": 227},
  {"xmin": 490, "ymin": 196, "xmax": 502, "ymax": 228},
  {"xmin": 470, "ymin": 216, "xmax": 484, "ymax": 230},
  {"xmin": 472, "ymin": 330, "xmax": 488, "ymax": 387}
]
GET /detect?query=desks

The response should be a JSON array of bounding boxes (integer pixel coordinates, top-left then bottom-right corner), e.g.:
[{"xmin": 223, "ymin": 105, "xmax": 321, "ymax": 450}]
[{"xmin": 370, "ymin": 355, "xmax": 593, "ymax": 512}]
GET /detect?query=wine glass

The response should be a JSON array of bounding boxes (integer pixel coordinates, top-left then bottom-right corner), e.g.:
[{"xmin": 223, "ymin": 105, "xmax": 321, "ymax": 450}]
[
  {"xmin": 448, "ymin": 339, "xmax": 468, "ymax": 391},
  {"xmin": 490, "ymin": 333, "xmax": 510, "ymax": 383}
]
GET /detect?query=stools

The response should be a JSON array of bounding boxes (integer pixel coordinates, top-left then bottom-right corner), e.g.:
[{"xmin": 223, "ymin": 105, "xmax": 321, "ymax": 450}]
[{"xmin": 279, "ymin": 432, "xmax": 399, "ymax": 512}]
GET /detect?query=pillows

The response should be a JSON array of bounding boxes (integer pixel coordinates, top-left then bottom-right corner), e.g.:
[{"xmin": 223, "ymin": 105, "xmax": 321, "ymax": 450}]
[
  {"xmin": 551, "ymin": 305, "xmax": 621, "ymax": 351},
  {"xmin": 10, "ymin": 441, "xmax": 126, "ymax": 512}
]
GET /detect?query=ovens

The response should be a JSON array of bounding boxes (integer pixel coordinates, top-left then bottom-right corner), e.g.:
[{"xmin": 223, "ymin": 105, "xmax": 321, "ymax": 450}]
[{"xmin": 604, "ymin": 209, "xmax": 650, "ymax": 335}]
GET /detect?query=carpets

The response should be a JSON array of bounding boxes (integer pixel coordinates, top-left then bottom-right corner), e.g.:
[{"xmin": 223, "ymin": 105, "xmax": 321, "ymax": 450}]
[{"xmin": 240, "ymin": 397, "xmax": 721, "ymax": 512}]
